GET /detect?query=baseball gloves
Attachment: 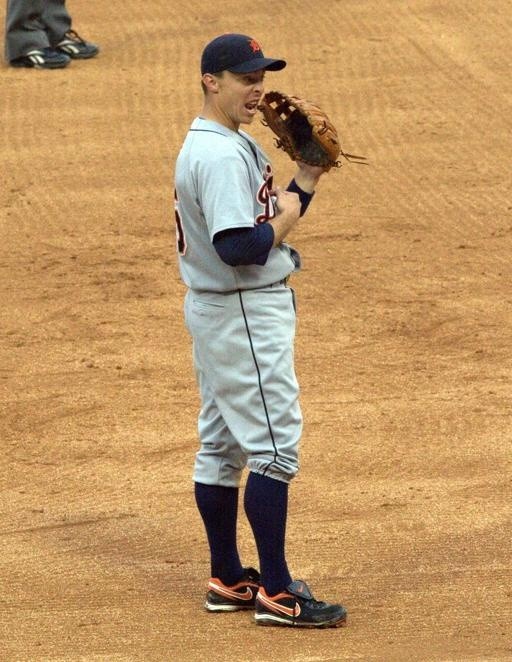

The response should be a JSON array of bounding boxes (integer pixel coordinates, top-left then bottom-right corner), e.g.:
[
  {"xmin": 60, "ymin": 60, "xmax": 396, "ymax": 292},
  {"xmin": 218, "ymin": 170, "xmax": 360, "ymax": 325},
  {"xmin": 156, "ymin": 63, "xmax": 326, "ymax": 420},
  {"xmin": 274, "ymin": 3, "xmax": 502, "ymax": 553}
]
[{"xmin": 256, "ymin": 91, "xmax": 339, "ymax": 171}]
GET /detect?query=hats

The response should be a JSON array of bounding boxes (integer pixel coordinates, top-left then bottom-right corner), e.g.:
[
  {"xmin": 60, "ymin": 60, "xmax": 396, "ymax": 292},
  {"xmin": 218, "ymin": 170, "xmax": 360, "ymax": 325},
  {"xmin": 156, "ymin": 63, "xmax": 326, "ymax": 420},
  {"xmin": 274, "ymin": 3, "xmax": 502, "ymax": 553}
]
[{"xmin": 201, "ymin": 34, "xmax": 286, "ymax": 75}]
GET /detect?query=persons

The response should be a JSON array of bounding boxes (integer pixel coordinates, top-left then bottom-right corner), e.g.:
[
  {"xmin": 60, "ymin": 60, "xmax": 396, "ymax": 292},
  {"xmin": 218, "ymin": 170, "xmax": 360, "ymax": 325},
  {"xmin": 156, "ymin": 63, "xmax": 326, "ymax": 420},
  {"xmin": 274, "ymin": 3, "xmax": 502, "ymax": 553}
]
[
  {"xmin": 6, "ymin": 1, "xmax": 99, "ymax": 70},
  {"xmin": 173, "ymin": 33, "xmax": 347, "ymax": 628}
]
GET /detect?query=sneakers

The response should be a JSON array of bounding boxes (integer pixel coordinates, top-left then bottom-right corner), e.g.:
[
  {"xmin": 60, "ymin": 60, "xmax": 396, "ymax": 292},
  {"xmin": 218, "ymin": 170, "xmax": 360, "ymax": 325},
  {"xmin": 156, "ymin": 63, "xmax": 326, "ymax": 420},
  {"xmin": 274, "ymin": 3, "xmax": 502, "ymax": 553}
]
[
  {"xmin": 9, "ymin": 46, "xmax": 71, "ymax": 68},
  {"xmin": 255, "ymin": 582, "xmax": 347, "ymax": 627},
  {"xmin": 205, "ymin": 568, "xmax": 261, "ymax": 612},
  {"xmin": 53, "ymin": 35, "xmax": 98, "ymax": 59}
]
